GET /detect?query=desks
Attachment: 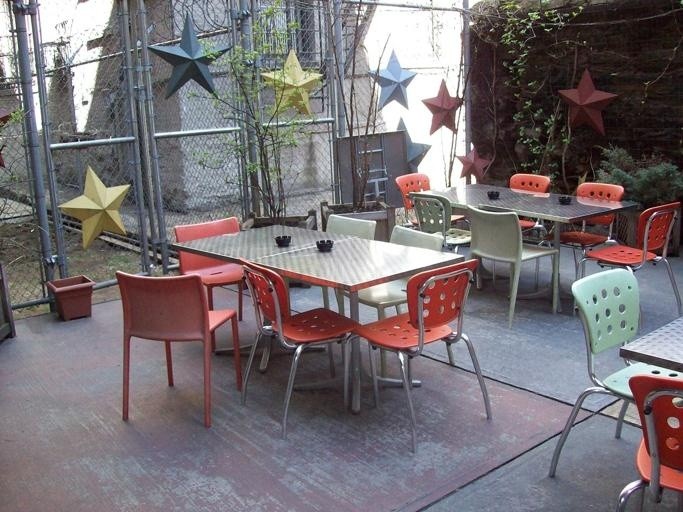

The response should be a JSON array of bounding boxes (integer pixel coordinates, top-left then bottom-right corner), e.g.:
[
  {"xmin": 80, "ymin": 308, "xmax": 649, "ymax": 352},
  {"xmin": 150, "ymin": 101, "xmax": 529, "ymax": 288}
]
[
  {"xmin": 619, "ymin": 315, "xmax": 681, "ymax": 374},
  {"xmin": 411, "ymin": 184, "xmax": 639, "ymax": 316}
]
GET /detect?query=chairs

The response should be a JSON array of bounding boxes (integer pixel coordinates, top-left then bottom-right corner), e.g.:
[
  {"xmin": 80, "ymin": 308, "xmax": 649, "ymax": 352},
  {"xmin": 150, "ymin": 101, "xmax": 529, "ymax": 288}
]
[
  {"xmin": 340, "ymin": 225, "xmax": 445, "ymax": 377},
  {"xmin": 510, "ymin": 174, "xmax": 554, "ymax": 261},
  {"xmin": 408, "ymin": 192, "xmax": 470, "ymax": 253},
  {"xmin": 395, "ymin": 172, "xmax": 471, "ymax": 229},
  {"xmin": 573, "ymin": 201, "xmax": 683, "ymax": 315},
  {"xmin": 171, "ymin": 224, "xmax": 465, "ymax": 414},
  {"xmin": 357, "ymin": 259, "xmax": 492, "ymax": 453},
  {"xmin": 116, "ymin": 271, "xmax": 242, "ymax": 428},
  {"xmin": 322, "ymin": 216, "xmax": 378, "ymax": 311},
  {"xmin": 174, "ymin": 217, "xmax": 248, "ymax": 350},
  {"xmin": 546, "ymin": 267, "xmax": 682, "ymax": 477},
  {"xmin": 466, "ymin": 205, "xmax": 561, "ymax": 330},
  {"xmin": 237, "ymin": 257, "xmax": 364, "ymax": 438},
  {"xmin": 614, "ymin": 375, "xmax": 682, "ymax": 512},
  {"xmin": 536, "ymin": 181, "xmax": 626, "ymax": 288}
]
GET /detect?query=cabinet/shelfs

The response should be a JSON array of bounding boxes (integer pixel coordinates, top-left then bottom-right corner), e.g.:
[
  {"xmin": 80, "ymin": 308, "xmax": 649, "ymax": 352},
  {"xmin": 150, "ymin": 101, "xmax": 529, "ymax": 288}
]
[{"xmin": 0, "ymin": 261, "xmax": 16, "ymax": 343}]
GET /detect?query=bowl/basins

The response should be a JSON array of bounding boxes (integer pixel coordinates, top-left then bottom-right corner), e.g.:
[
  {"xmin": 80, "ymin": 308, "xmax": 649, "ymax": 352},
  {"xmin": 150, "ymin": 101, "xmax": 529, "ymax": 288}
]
[
  {"xmin": 274, "ymin": 235, "xmax": 292, "ymax": 247},
  {"xmin": 558, "ymin": 195, "xmax": 573, "ymax": 206},
  {"xmin": 486, "ymin": 190, "xmax": 500, "ymax": 199},
  {"xmin": 314, "ymin": 239, "xmax": 333, "ymax": 251}
]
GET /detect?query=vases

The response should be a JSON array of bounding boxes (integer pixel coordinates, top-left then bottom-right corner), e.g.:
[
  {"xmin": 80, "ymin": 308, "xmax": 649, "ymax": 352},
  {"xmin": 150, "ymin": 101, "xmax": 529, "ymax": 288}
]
[{"xmin": 46, "ymin": 276, "xmax": 97, "ymax": 321}]
